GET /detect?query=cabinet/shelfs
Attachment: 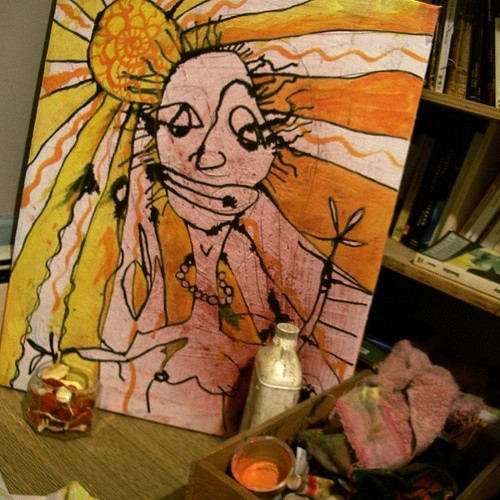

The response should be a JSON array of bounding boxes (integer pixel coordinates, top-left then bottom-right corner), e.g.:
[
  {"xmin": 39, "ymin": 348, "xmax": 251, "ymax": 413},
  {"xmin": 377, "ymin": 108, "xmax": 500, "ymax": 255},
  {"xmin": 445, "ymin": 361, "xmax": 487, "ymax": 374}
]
[{"xmin": 357, "ymin": 0, "xmax": 500, "ymax": 449}]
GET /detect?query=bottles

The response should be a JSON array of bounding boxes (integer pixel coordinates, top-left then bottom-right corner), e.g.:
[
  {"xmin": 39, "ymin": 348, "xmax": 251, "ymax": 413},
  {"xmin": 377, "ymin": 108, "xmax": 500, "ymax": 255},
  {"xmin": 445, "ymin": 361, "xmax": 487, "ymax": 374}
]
[
  {"xmin": 236, "ymin": 321, "xmax": 304, "ymax": 437},
  {"xmin": 290, "ymin": 474, "xmax": 343, "ymax": 500}
]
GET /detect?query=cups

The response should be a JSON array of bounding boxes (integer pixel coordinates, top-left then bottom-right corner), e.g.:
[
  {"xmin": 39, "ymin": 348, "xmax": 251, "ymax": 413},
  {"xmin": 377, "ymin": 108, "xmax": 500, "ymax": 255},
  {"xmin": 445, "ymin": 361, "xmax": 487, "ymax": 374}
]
[{"xmin": 229, "ymin": 436, "xmax": 295, "ymax": 497}]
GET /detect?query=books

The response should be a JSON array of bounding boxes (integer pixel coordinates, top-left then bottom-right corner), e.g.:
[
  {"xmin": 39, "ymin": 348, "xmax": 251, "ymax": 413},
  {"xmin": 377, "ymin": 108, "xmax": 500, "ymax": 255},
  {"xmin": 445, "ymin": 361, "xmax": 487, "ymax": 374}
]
[
  {"xmin": 414, "ymin": 229, "xmax": 500, "ymax": 301},
  {"xmin": 386, "ymin": 0, "xmax": 499, "ymax": 247}
]
